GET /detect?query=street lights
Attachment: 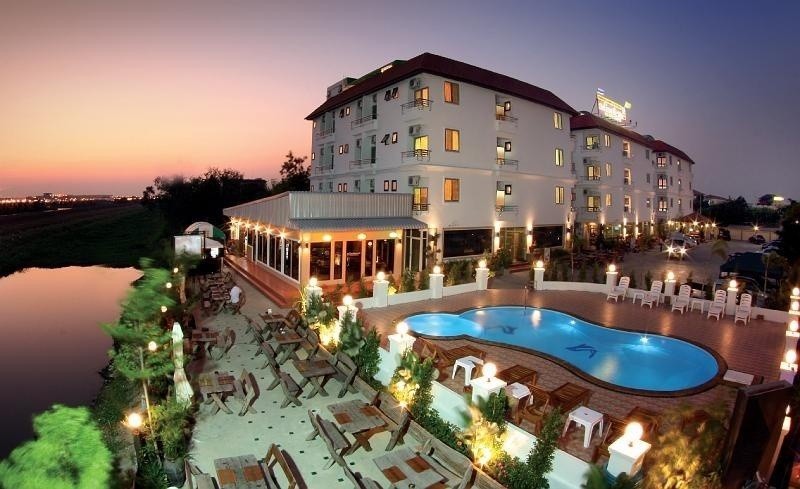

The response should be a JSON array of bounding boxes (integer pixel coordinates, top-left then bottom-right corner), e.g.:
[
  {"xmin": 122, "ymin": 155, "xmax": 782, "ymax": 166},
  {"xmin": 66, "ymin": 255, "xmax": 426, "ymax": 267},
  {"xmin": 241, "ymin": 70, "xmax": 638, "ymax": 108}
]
[{"xmin": 135, "ymin": 342, "xmax": 163, "ymax": 467}]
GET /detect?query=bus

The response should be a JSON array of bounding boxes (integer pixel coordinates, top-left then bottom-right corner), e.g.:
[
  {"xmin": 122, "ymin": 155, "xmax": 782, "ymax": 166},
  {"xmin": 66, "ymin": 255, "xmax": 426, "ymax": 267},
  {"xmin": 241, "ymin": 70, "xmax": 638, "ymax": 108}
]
[{"xmin": 667, "ymin": 231, "xmax": 685, "ymax": 259}]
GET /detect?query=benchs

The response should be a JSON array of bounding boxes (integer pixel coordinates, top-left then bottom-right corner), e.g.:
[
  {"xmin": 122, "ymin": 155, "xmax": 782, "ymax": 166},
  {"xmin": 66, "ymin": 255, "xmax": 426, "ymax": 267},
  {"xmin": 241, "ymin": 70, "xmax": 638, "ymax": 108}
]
[
  {"xmin": 185, "ymin": 367, "xmax": 257, "ymax": 416},
  {"xmin": 343, "ymin": 465, "xmax": 379, "ymax": 488},
  {"xmin": 241, "ymin": 308, "xmax": 359, "ymax": 409},
  {"xmin": 184, "ymin": 270, "xmax": 247, "ymax": 317},
  {"xmin": 183, "ymin": 325, "xmax": 237, "ymax": 362},
  {"xmin": 184, "ymin": 459, "xmax": 216, "ymax": 488},
  {"xmin": 419, "ymin": 440, "xmax": 475, "ymax": 488},
  {"xmin": 369, "ymin": 390, "xmax": 411, "ymax": 451},
  {"xmin": 308, "ymin": 409, "xmax": 348, "ymax": 470},
  {"xmin": 259, "ymin": 442, "xmax": 296, "ymax": 488}
]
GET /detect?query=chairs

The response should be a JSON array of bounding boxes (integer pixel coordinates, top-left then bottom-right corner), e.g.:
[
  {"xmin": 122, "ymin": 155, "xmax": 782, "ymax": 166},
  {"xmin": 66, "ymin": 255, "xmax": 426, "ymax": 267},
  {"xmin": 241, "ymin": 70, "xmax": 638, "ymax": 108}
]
[
  {"xmin": 606, "ymin": 276, "xmax": 753, "ymax": 327},
  {"xmin": 418, "ymin": 336, "xmax": 663, "ymax": 465}
]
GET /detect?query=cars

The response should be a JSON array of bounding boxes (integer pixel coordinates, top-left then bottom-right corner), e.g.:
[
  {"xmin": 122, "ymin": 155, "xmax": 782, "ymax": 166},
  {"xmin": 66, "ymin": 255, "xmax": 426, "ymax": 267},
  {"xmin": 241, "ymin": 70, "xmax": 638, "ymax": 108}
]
[
  {"xmin": 719, "ymin": 228, "xmax": 731, "ymax": 241},
  {"xmin": 684, "ymin": 232, "xmax": 701, "ymax": 249},
  {"xmin": 749, "ymin": 235, "xmax": 781, "ymax": 253}
]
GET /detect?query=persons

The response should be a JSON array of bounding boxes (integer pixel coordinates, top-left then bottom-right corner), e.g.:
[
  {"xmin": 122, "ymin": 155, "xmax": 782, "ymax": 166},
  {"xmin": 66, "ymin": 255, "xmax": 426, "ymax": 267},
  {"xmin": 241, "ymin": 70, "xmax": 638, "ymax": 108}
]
[{"xmin": 228, "ymin": 282, "xmax": 244, "ymax": 304}]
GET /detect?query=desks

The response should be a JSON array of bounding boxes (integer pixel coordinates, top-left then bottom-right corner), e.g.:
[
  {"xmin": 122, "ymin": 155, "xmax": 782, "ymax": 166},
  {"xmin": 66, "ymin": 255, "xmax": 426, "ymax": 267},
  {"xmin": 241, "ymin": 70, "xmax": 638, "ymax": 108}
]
[
  {"xmin": 212, "ymin": 454, "xmax": 267, "ymax": 488},
  {"xmin": 326, "ymin": 399, "xmax": 386, "ymax": 456},
  {"xmin": 371, "ymin": 446, "xmax": 445, "ymax": 488}
]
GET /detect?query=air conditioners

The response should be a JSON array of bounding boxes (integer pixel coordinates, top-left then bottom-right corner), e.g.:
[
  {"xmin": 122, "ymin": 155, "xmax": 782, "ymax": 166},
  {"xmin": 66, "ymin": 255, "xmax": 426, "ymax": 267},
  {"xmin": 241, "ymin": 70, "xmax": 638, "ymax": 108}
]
[
  {"xmin": 354, "ymin": 77, "xmax": 420, "ymax": 188},
  {"xmin": 583, "ymin": 156, "xmax": 592, "ymax": 165}
]
